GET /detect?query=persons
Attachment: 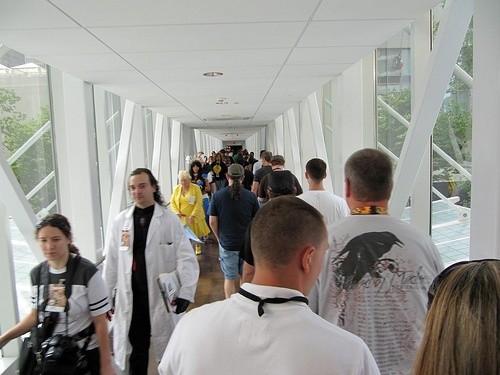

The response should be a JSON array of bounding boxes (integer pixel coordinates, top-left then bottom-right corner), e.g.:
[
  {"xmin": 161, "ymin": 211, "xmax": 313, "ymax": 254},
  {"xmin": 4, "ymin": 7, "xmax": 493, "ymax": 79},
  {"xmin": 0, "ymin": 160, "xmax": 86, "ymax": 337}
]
[
  {"xmin": 101, "ymin": 167, "xmax": 199, "ymax": 375},
  {"xmin": 307, "ymin": 147, "xmax": 444, "ymax": 374},
  {"xmin": 0, "ymin": 213, "xmax": 115, "ymax": 374},
  {"xmin": 170, "ymin": 147, "xmax": 350, "ymax": 298},
  {"xmin": 156, "ymin": 195, "xmax": 381, "ymax": 375},
  {"xmin": 414, "ymin": 258, "xmax": 500, "ymax": 374}
]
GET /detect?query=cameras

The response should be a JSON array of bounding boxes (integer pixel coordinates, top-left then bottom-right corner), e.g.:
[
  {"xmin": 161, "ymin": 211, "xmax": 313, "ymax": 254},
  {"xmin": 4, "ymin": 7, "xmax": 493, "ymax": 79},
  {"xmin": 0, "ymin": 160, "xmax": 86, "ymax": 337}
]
[{"xmin": 40, "ymin": 334, "xmax": 80, "ymax": 368}]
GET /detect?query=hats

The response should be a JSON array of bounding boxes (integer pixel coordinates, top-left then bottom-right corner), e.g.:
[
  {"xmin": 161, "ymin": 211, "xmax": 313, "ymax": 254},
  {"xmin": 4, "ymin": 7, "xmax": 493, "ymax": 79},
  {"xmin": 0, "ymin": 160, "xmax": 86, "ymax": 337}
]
[
  {"xmin": 228, "ymin": 163, "xmax": 244, "ymax": 176},
  {"xmin": 270, "ymin": 169, "xmax": 295, "ymax": 195}
]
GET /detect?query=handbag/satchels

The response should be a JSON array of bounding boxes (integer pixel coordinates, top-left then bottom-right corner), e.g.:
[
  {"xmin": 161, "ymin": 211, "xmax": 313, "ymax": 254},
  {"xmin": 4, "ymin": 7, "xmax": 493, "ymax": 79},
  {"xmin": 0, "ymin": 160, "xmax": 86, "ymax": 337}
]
[{"xmin": 18, "ymin": 337, "xmax": 34, "ymax": 372}]
